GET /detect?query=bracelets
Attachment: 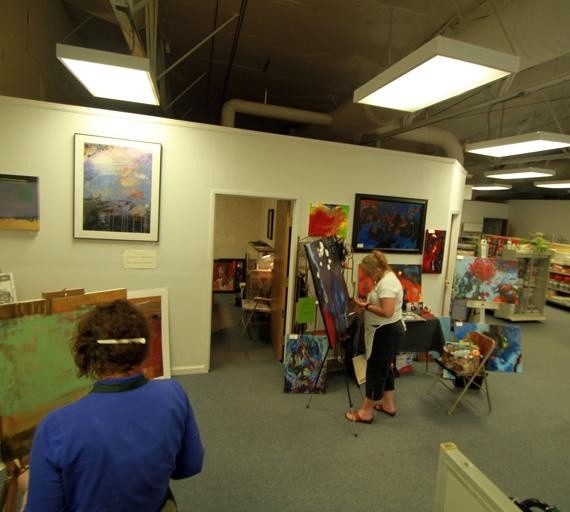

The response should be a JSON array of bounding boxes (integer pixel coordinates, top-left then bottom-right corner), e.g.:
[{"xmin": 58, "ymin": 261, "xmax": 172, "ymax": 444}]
[{"xmin": 364, "ymin": 303, "xmax": 372, "ymax": 309}]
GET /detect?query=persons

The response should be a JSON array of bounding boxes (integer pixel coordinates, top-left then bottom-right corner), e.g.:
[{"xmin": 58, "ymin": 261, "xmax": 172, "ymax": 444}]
[
  {"xmin": 212, "ymin": 264, "xmax": 233, "ymax": 291},
  {"xmin": 344, "ymin": 250, "xmax": 409, "ymax": 423},
  {"xmin": 24, "ymin": 299, "xmax": 204, "ymax": 512}
]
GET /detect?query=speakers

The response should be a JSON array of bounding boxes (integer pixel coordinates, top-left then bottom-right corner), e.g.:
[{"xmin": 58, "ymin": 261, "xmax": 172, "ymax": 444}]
[{"xmin": 483, "ymin": 217, "xmax": 508, "ymax": 236}]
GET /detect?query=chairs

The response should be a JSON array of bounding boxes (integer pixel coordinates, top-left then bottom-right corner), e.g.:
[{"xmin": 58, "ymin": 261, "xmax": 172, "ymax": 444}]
[
  {"xmin": 237, "ymin": 278, "xmax": 279, "ymax": 339},
  {"xmin": 424, "ymin": 328, "xmax": 497, "ymax": 416}
]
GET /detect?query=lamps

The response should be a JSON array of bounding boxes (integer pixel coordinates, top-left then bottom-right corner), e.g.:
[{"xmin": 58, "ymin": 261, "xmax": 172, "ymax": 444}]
[
  {"xmin": 351, "ymin": 3, "xmax": 521, "ymax": 118},
  {"xmin": 54, "ymin": 0, "xmax": 161, "ymax": 108},
  {"xmin": 463, "ymin": 93, "xmax": 570, "ymax": 192}
]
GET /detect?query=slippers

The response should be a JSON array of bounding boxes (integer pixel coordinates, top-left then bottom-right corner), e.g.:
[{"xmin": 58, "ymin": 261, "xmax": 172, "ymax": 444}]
[
  {"xmin": 375, "ymin": 405, "xmax": 395, "ymax": 416},
  {"xmin": 346, "ymin": 411, "xmax": 372, "ymax": 423}
]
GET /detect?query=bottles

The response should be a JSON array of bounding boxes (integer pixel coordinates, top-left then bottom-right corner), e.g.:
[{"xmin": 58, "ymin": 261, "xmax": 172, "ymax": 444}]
[{"xmin": 479, "ymin": 239, "xmax": 489, "ymax": 259}]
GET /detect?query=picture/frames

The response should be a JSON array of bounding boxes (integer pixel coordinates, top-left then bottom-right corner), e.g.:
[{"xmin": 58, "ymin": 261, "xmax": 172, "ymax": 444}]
[
  {"xmin": 349, "ymin": 192, "xmax": 429, "ymax": 256},
  {"xmin": 212, "ymin": 258, "xmax": 238, "ymax": 293},
  {"xmin": 70, "ymin": 129, "xmax": 165, "ymax": 244},
  {"xmin": 124, "ymin": 285, "xmax": 173, "ymax": 383}
]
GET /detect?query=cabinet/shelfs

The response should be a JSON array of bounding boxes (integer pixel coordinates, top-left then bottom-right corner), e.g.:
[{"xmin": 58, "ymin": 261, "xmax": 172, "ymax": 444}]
[
  {"xmin": 244, "ymin": 241, "xmax": 276, "ymax": 286},
  {"xmin": 547, "ymin": 240, "xmax": 570, "ymax": 311},
  {"xmin": 494, "ymin": 242, "xmax": 555, "ymax": 324}
]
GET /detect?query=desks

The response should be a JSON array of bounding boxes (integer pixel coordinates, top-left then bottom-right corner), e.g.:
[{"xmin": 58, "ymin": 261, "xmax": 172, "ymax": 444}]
[{"xmin": 390, "ymin": 307, "xmax": 445, "ymax": 376}]
